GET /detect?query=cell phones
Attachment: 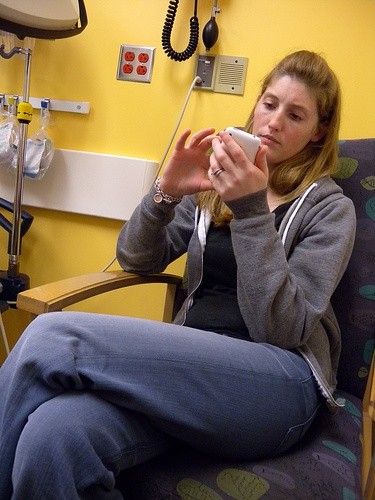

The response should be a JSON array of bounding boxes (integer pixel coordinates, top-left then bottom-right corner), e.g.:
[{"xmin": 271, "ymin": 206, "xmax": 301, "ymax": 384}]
[{"xmin": 222, "ymin": 127, "xmax": 261, "ymax": 165}]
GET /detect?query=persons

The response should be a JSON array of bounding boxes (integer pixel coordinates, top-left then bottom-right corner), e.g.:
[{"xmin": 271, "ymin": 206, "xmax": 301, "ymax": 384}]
[{"xmin": 1, "ymin": 50, "xmax": 356, "ymax": 500}]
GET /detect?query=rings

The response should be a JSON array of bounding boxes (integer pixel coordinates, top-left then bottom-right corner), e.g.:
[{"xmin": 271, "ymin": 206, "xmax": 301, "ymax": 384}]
[
  {"xmin": 207, "ymin": 169, "xmax": 213, "ymax": 177},
  {"xmin": 213, "ymin": 167, "xmax": 224, "ymax": 176}
]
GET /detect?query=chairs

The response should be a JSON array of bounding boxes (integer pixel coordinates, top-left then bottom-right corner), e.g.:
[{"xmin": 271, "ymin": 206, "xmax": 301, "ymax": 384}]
[{"xmin": 16, "ymin": 138, "xmax": 375, "ymax": 500}]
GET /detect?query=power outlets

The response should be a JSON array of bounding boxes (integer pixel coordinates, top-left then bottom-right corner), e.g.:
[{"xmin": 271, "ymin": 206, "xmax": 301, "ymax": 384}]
[
  {"xmin": 116, "ymin": 44, "xmax": 156, "ymax": 84},
  {"xmin": 193, "ymin": 54, "xmax": 218, "ymax": 90}
]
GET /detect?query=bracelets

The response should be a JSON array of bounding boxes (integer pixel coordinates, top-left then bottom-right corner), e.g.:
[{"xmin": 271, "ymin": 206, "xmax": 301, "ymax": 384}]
[{"xmin": 153, "ymin": 176, "xmax": 184, "ymax": 204}]
